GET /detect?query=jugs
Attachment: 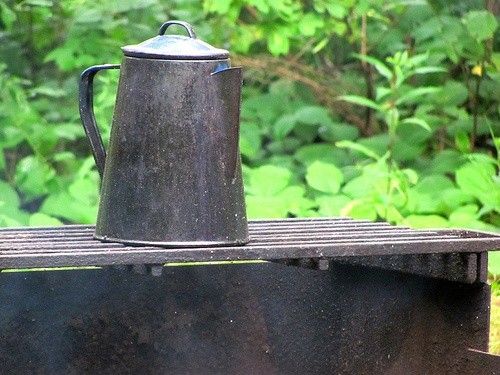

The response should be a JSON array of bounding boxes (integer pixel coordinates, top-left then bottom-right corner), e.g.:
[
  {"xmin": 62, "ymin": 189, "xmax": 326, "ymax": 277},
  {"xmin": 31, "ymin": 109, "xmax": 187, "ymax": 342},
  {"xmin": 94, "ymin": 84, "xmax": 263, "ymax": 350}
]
[{"xmin": 77, "ymin": 18, "xmax": 253, "ymax": 248}]
